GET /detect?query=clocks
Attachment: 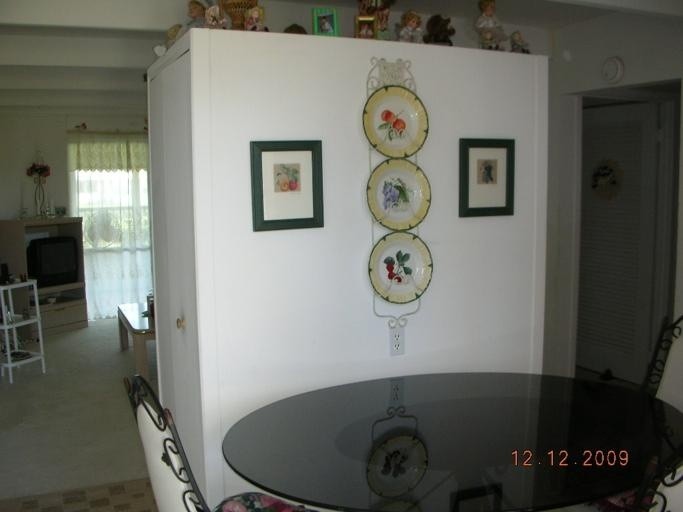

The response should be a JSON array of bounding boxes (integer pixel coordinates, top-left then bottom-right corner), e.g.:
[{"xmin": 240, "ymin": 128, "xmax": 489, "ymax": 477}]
[{"xmin": 601, "ymin": 56, "xmax": 624, "ymax": 84}]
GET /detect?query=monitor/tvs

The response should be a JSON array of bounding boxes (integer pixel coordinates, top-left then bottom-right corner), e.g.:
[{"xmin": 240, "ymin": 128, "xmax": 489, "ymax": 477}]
[{"xmin": 27, "ymin": 236, "xmax": 79, "ymax": 289}]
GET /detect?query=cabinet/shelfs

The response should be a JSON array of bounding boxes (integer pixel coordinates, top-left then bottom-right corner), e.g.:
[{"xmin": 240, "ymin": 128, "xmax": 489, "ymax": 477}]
[
  {"xmin": 1, "ymin": 279, "xmax": 47, "ymax": 384},
  {"xmin": 1, "ymin": 214, "xmax": 88, "ymax": 341}
]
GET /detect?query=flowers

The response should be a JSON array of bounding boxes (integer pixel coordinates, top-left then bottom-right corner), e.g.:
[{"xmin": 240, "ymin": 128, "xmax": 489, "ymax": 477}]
[{"xmin": 27, "ymin": 161, "xmax": 52, "ymax": 178}]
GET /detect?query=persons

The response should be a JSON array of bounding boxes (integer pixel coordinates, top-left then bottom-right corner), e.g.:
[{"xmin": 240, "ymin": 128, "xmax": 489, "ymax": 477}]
[
  {"xmin": 396, "ymin": 11, "xmax": 425, "ymax": 46},
  {"xmin": 469, "ymin": 0, "xmax": 508, "ymax": 44},
  {"xmin": 199, "ymin": 3, "xmax": 232, "ymax": 31},
  {"xmin": 241, "ymin": 6, "xmax": 272, "ymax": 33},
  {"xmin": 416, "ymin": 14, "xmax": 456, "ymax": 47},
  {"xmin": 151, "ymin": 23, "xmax": 184, "ymax": 57},
  {"xmin": 320, "ymin": 17, "xmax": 333, "ymax": 32},
  {"xmin": 509, "ymin": 29, "xmax": 531, "ymax": 55},
  {"xmin": 173, "ymin": 0, "xmax": 217, "ymax": 44},
  {"xmin": 359, "ymin": 23, "xmax": 375, "ymax": 37}
]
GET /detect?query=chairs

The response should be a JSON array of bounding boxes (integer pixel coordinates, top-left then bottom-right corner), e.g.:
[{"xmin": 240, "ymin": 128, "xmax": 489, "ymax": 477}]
[
  {"xmin": 122, "ymin": 371, "xmax": 311, "ymax": 512},
  {"xmin": 572, "ymin": 310, "xmax": 683, "ymax": 511}
]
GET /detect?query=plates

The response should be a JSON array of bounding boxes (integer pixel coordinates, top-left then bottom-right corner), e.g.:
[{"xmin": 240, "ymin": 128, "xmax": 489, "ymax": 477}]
[
  {"xmin": 367, "ymin": 158, "xmax": 431, "ymax": 230},
  {"xmin": 366, "ymin": 432, "xmax": 430, "ymax": 497},
  {"xmin": 362, "ymin": 85, "xmax": 429, "ymax": 159},
  {"xmin": 368, "ymin": 231, "xmax": 434, "ymax": 304}
]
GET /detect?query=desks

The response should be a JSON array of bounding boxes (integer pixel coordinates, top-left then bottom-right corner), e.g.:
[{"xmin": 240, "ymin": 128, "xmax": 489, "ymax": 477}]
[
  {"xmin": 222, "ymin": 372, "xmax": 681, "ymax": 511},
  {"xmin": 117, "ymin": 302, "xmax": 156, "ymax": 382}
]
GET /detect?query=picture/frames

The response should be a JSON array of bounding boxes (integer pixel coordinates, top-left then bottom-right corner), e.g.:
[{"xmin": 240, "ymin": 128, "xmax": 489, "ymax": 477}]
[
  {"xmin": 458, "ymin": 137, "xmax": 515, "ymax": 217},
  {"xmin": 250, "ymin": 140, "xmax": 324, "ymax": 232}
]
